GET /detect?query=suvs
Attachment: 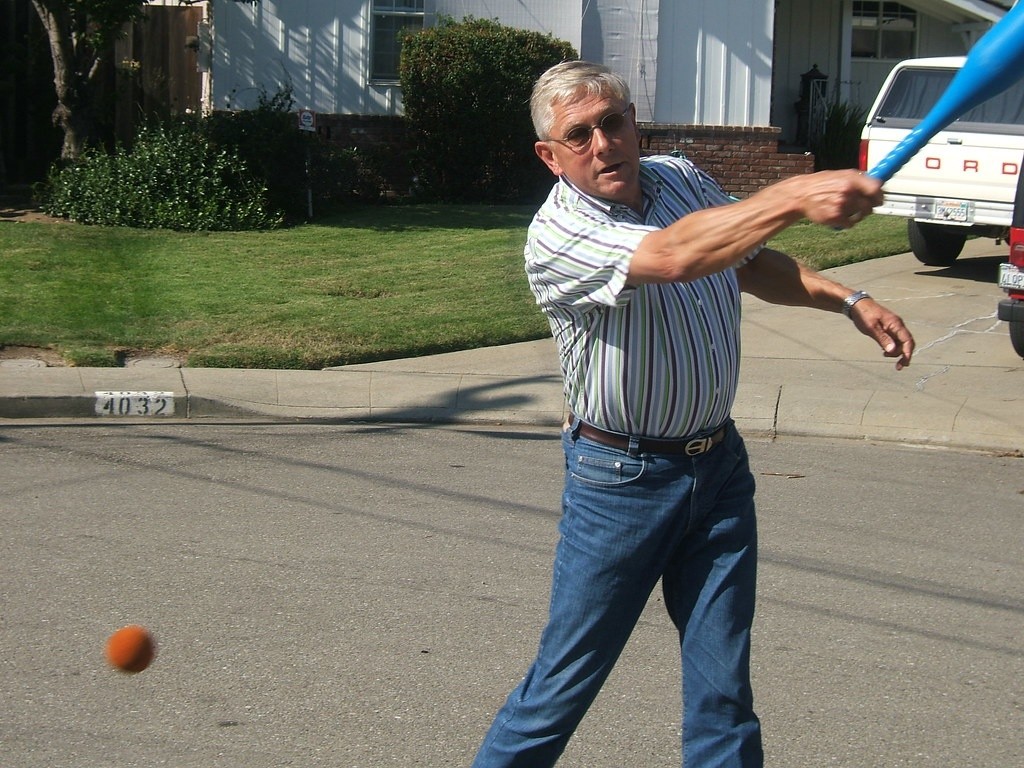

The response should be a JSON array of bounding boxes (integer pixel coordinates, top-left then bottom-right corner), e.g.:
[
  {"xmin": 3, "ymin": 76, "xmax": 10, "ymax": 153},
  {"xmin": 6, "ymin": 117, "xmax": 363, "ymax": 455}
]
[{"xmin": 857, "ymin": 54, "xmax": 1023, "ymax": 360}]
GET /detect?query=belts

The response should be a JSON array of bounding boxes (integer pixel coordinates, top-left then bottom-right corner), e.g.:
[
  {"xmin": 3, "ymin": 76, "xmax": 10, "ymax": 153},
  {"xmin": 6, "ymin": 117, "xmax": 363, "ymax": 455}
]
[{"xmin": 568, "ymin": 412, "xmax": 728, "ymax": 458}]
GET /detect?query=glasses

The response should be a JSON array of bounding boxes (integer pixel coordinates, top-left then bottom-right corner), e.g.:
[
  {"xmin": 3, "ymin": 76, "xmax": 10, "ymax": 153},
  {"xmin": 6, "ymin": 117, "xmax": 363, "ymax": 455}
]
[{"xmin": 545, "ymin": 104, "xmax": 631, "ymax": 147}]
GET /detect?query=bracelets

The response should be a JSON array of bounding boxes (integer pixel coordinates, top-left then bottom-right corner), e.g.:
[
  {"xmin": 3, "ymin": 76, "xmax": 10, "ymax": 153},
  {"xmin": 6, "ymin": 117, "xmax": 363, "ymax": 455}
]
[{"xmin": 840, "ymin": 290, "xmax": 871, "ymax": 319}]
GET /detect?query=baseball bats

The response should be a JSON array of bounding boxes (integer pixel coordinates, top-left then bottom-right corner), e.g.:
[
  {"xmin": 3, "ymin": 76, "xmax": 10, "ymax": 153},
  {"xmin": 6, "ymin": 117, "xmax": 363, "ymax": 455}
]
[{"xmin": 830, "ymin": 1, "xmax": 1024, "ymax": 234}]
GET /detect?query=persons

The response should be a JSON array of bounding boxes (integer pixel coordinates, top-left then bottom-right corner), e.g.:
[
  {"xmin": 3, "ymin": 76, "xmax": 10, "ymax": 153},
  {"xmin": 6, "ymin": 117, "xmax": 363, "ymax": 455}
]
[{"xmin": 475, "ymin": 59, "xmax": 916, "ymax": 768}]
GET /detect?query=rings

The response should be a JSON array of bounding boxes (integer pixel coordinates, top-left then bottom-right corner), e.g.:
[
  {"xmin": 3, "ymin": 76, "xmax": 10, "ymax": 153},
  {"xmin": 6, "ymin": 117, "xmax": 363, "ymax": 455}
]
[{"xmin": 848, "ymin": 210, "xmax": 863, "ymax": 221}]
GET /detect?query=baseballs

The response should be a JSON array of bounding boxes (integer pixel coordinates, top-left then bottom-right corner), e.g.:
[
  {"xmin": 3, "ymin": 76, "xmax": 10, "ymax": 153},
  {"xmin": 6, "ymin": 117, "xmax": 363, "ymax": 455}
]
[{"xmin": 107, "ymin": 627, "xmax": 155, "ymax": 674}]
